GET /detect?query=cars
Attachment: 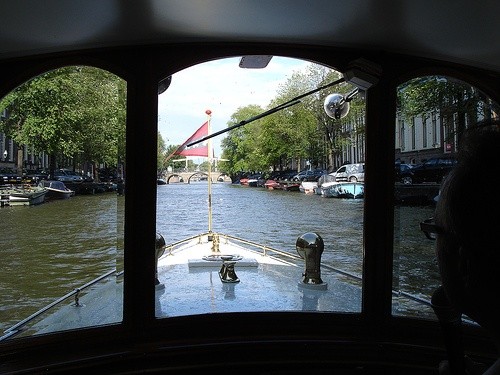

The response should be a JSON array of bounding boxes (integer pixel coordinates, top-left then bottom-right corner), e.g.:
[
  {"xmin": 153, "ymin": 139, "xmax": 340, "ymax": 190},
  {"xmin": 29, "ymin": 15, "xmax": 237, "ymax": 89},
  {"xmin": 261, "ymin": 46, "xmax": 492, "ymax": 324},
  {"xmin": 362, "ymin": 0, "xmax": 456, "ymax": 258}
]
[
  {"xmin": 0, "ymin": 167, "xmax": 94, "ymax": 183},
  {"xmin": 248, "ymin": 158, "xmax": 414, "ymax": 184},
  {"xmin": 410, "ymin": 156, "xmax": 459, "ymax": 183}
]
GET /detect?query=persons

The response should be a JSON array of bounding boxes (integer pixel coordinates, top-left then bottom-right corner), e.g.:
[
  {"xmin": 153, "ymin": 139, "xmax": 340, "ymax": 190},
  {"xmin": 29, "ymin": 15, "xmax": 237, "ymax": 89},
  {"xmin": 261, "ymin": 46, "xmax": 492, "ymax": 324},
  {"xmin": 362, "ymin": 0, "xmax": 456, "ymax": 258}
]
[{"xmin": 420, "ymin": 119, "xmax": 500, "ymax": 375}]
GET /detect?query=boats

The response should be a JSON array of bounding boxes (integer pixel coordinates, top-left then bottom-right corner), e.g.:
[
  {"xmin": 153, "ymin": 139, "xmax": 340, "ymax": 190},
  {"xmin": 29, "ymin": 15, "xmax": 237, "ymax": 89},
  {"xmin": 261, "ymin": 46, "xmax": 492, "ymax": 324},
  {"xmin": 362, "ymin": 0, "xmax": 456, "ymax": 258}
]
[
  {"xmin": 0, "ymin": 182, "xmax": 117, "ymax": 206},
  {"xmin": 323, "ymin": 182, "xmax": 364, "ymax": 198},
  {"xmin": 240, "ymin": 179, "xmax": 339, "ymax": 194}
]
